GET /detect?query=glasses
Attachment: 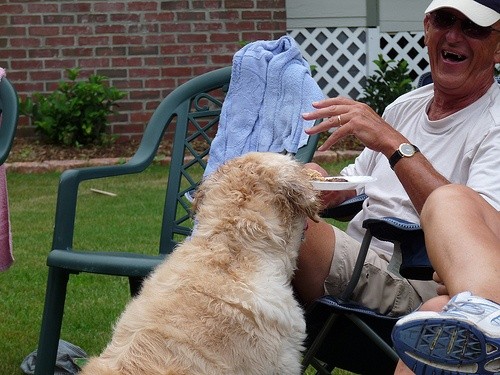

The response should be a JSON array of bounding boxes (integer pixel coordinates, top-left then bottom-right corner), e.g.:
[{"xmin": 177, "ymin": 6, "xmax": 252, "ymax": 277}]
[{"xmin": 430, "ymin": 12, "xmax": 500, "ymax": 39}]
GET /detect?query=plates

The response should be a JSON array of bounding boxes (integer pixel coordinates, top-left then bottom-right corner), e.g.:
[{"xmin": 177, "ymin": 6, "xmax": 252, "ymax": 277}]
[{"xmin": 310, "ymin": 176, "xmax": 378, "ymax": 190}]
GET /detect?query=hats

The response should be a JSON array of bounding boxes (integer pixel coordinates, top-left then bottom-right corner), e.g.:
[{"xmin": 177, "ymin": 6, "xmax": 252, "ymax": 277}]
[{"xmin": 423, "ymin": 0, "xmax": 500, "ymax": 28}]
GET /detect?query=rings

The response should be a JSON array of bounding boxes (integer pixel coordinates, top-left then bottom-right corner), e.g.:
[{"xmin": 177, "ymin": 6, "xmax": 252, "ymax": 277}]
[{"xmin": 337, "ymin": 114, "xmax": 342, "ymax": 125}]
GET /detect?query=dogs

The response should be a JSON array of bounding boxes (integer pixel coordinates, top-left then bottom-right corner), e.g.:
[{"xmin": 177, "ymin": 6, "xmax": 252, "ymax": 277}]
[{"xmin": 74, "ymin": 152, "xmax": 324, "ymax": 375}]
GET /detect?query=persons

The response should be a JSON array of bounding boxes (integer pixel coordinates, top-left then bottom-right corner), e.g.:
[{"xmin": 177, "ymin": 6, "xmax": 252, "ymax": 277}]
[
  {"xmin": 390, "ymin": 185, "xmax": 500, "ymax": 375},
  {"xmin": 237, "ymin": 0, "xmax": 500, "ymax": 319}
]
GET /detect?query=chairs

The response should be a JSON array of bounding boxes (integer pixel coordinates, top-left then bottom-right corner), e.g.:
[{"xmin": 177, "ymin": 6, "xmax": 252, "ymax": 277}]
[
  {"xmin": 293, "ymin": 195, "xmax": 438, "ymax": 375},
  {"xmin": 0, "ymin": 75, "xmax": 20, "ymax": 273},
  {"xmin": 35, "ymin": 67, "xmax": 326, "ymax": 375}
]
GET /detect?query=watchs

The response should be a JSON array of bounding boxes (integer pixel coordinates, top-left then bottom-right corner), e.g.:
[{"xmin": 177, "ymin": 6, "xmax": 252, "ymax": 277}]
[{"xmin": 388, "ymin": 143, "xmax": 420, "ymax": 170}]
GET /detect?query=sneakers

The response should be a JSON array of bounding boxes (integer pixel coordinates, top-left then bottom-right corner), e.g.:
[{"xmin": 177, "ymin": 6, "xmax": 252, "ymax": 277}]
[{"xmin": 390, "ymin": 290, "xmax": 500, "ymax": 375}]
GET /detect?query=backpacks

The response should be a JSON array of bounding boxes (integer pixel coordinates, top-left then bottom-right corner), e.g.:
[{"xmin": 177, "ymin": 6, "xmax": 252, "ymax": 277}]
[{"xmin": 21, "ymin": 339, "xmax": 86, "ymax": 375}]
